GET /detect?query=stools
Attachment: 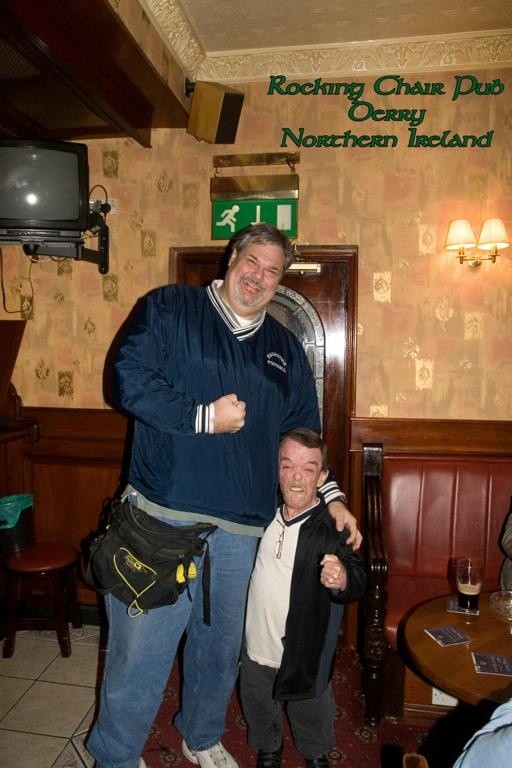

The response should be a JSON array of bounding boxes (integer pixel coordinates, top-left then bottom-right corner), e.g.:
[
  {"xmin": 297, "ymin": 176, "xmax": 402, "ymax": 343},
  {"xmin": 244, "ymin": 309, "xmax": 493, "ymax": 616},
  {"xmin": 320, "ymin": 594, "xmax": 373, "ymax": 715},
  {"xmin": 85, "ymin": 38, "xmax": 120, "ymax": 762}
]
[{"xmin": 2, "ymin": 544, "xmax": 85, "ymax": 659}]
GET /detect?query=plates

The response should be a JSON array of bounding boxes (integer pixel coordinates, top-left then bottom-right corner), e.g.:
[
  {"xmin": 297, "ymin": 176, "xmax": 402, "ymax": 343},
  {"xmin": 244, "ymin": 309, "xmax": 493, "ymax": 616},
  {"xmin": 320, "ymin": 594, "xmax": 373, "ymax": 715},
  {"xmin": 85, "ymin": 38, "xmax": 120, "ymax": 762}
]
[{"xmin": 488, "ymin": 591, "xmax": 512, "ymax": 624}]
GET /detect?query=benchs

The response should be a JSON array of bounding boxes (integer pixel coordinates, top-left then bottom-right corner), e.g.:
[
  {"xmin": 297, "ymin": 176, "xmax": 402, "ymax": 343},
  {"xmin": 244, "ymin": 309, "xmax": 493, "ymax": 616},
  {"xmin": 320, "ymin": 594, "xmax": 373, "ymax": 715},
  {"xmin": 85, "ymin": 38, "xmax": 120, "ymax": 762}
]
[{"xmin": 353, "ymin": 439, "xmax": 510, "ymax": 728}]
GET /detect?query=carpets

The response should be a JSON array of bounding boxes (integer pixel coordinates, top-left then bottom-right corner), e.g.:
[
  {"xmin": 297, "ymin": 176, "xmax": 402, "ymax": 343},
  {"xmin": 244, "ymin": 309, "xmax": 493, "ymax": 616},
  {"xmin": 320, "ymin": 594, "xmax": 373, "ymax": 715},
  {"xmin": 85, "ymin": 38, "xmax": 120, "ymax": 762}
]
[{"xmin": 135, "ymin": 634, "xmax": 437, "ymax": 767}]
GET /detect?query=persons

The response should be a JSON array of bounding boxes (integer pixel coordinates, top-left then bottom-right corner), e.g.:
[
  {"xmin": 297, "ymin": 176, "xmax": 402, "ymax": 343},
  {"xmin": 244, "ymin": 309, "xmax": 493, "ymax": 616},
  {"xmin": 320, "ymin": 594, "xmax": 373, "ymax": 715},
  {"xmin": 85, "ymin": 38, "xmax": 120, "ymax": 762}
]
[
  {"xmin": 84, "ymin": 227, "xmax": 363, "ymax": 768},
  {"xmin": 236, "ymin": 428, "xmax": 367, "ymax": 768}
]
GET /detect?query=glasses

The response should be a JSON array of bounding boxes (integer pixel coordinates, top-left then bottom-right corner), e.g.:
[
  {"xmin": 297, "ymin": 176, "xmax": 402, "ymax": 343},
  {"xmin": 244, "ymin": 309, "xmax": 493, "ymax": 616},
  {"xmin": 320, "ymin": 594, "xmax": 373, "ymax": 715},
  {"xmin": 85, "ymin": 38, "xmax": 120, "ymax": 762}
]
[{"xmin": 274, "ymin": 520, "xmax": 286, "ymax": 559}]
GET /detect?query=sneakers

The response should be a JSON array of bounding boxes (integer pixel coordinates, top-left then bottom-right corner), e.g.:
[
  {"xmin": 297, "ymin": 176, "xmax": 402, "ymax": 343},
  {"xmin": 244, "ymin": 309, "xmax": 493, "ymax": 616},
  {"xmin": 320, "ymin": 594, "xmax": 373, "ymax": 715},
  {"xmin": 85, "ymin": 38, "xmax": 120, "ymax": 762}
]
[{"xmin": 182, "ymin": 738, "xmax": 240, "ymax": 768}]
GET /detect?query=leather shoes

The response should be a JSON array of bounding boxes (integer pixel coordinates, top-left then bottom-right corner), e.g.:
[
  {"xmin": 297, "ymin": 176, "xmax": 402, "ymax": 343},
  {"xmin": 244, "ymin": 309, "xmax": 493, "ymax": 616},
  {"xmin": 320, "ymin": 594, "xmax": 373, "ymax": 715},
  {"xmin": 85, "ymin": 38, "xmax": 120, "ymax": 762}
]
[
  {"xmin": 258, "ymin": 737, "xmax": 283, "ymax": 768},
  {"xmin": 306, "ymin": 756, "xmax": 329, "ymax": 768}
]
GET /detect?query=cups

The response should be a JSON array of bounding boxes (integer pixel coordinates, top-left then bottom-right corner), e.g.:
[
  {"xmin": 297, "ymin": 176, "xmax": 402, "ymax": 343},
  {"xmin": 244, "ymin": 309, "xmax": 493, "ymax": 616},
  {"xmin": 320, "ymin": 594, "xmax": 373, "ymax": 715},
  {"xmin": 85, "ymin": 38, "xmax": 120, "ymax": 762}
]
[{"xmin": 455, "ymin": 556, "xmax": 484, "ymax": 611}]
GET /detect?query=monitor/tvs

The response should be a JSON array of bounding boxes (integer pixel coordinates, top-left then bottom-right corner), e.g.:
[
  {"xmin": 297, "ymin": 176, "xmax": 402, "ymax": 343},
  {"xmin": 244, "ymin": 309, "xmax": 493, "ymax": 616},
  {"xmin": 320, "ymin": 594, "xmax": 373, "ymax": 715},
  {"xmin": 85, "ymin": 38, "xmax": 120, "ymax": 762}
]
[{"xmin": 1, "ymin": 138, "xmax": 89, "ymax": 232}]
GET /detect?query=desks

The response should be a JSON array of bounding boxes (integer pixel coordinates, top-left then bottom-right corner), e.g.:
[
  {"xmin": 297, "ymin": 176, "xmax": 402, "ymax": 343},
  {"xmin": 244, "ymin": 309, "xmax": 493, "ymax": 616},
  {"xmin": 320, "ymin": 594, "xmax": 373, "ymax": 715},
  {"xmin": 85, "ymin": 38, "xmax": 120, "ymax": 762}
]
[{"xmin": 399, "ymin": 589, "xmax": 512, "ymax": 713}]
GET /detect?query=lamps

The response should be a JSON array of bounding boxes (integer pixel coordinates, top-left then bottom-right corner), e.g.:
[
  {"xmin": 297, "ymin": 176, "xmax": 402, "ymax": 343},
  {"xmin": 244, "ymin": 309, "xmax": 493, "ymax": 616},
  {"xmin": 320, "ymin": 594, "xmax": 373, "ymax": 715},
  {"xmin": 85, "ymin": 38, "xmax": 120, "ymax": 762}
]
[{"xmin": 443, "ymin": 216, "xmax": 509, "ymax": 271}]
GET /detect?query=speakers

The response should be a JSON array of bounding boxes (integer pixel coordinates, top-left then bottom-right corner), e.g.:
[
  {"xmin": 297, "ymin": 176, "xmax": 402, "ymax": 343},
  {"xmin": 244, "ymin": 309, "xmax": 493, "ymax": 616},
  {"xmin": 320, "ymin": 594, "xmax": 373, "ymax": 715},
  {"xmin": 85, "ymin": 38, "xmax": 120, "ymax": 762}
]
[{"xmin": 186, "ymin": 81, "xmax": 244, "ymax": 144}]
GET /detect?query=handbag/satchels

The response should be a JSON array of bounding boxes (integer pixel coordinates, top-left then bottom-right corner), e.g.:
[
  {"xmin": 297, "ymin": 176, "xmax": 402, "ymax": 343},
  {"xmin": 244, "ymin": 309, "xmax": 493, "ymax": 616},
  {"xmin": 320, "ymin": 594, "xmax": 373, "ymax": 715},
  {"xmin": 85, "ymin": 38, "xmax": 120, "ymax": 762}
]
[{"xmin": 88, "ymin": 500, "xmax": 214, "ymax": 609}]
